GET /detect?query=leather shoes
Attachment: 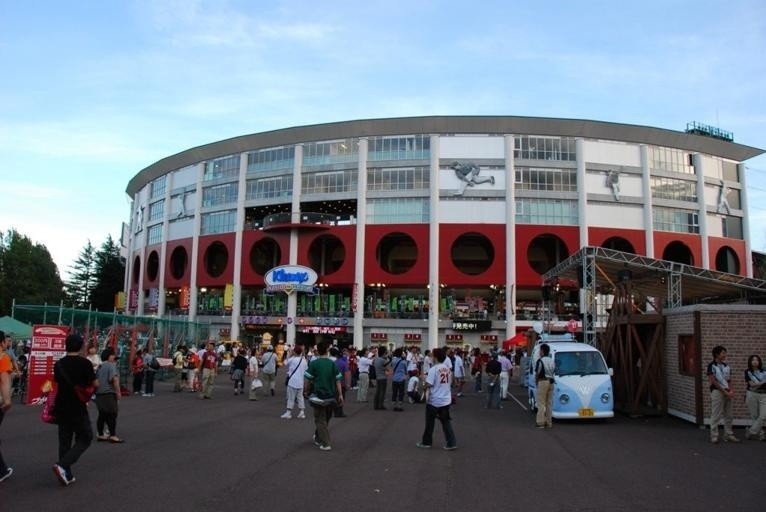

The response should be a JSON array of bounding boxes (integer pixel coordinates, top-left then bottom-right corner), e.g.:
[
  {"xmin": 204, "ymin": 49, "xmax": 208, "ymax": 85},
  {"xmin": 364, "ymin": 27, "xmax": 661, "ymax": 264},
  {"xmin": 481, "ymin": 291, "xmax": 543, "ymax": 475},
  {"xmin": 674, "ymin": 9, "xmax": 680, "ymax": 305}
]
[{"xmin": 97, "ymin": 433, "xmax": 124, "ymax": 444}]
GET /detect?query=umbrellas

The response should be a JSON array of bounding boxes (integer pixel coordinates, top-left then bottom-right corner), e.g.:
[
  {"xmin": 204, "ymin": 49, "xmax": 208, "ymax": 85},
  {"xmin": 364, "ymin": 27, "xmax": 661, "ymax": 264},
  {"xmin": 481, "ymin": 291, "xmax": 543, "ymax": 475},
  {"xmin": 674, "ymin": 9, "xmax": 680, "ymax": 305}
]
[{"xmin": 0, "ymin": 316, "xmax": 39, "ymax": 340}]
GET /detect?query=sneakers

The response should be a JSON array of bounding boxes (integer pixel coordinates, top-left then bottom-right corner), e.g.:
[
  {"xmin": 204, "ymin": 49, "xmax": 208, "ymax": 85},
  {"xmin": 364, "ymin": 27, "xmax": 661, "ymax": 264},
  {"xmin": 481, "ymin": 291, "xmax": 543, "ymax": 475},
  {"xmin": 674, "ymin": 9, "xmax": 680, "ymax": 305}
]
[
  {"xmin": 313, "ymin": 434, "xmax": 331, "ymax": 450},
  {"xmin": 297, "ymin": 415, "xmax": 305, "ymax": 419},
  {"xmin": 280, "ymin": 414, "xmax": 292, "ymax": 419},
  {"xmin": 0, "ymin": 468, "xmax": 12, "ymax": 481},
  {"xmin": 53, "ymin": 464, "xmax": 75, "ymax": 486}
]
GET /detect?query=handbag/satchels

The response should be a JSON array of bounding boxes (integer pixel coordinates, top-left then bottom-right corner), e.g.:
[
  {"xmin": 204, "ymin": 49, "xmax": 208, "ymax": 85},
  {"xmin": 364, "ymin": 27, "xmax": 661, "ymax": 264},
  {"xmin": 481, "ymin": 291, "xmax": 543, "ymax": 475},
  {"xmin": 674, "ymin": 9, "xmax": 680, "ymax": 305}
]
[
  {"xmin": 369, "ymin": 365, "xmax": 375, "ymax": 378},
  {"xmin": 183, "ymin": 362, "xmax": 194, "ymax": 369},
  {"xmin": 172, "ymin": 358, "xmax": 177, "ymax": 366},
  {"xmin": 42, "ymin": 387, "xmax": 57, "ymax": 423},
  {"xmin": 150, "ymin": 358, "xmax": 160, "ymax": 368},
  {"xmin": 285, "ymin": 375, "xmax": 289, "ymax": 385}
]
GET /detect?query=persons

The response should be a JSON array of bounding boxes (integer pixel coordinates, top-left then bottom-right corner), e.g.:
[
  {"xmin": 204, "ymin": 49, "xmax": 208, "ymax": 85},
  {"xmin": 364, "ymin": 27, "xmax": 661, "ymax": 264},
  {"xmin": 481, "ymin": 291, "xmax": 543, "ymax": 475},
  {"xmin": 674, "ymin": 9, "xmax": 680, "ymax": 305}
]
[
  {"xmin": 332, "ymin": 303, "xmax": 578, "ymax": 321},
  {"xmin": 533, "ymin": 344, "xmax": 557, "ymax": 429},
  {"xmin": 50, "ymin": 333, "xmax": 101, "ymax": 488},
  {"xmin": 218, "ymin": 341, "xmax": 278, "ymax": 401},
  {"xmin": 707, "ymin": 346, "xmax": 740, "ymax": 444},
  {"xmin": 85, "ymin": 343, "xmax": 102, "ymax": 374},
  {"xmin": 93, "ymin": 348, "xmax": 124, "ymax": 442},
  {"xmin": 278, "ymin": 341, "xmax": 431, "ymax": 419},
  {"xmin": 172, "ymin": 342, "xmax": 218, "ymax": 399},
  {"xmin": 416, "ymin": 348, "xmax": 458, "ymax": 451},
  {"xmin": 303, "ymin": 341, "xmax": 344, "ymax": 451},
  {"xmin": 131, "ymin": 347, "xmax": 158, "ymax": 396},
  {"xmin": 5, "ymin": 336, "xmax": 33, "ymax": 396},
  {"xmin": 744, "ymin": 353, "xmax": 766, "ymax": 441},
  {"xmin": 0, "ymin": 329, "xmax": 12, "ymax": 482},
  {"xmin": 441, "ymin": 346, "xmax": 527, "ymax": 410}
]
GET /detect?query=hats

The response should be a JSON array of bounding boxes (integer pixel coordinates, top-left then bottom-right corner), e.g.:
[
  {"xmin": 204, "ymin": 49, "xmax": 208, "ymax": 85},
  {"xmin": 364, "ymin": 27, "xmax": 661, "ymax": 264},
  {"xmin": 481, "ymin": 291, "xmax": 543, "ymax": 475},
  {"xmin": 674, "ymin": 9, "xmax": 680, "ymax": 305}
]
[
  {"xmin": 267, "ymin": 345, "xmax": 274, "ymax": 349},
  {"xmin": 294, "ymin": 347, "xmax": 302, "ymax": 352}
]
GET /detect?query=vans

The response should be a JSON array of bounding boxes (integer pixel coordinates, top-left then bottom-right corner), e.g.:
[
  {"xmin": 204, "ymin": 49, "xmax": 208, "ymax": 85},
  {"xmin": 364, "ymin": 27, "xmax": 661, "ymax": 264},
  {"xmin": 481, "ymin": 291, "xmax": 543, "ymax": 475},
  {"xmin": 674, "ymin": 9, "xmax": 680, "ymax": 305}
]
[{"xmin": 527, "ymin": 333, "xmax": 617, "ymax": 422}]
